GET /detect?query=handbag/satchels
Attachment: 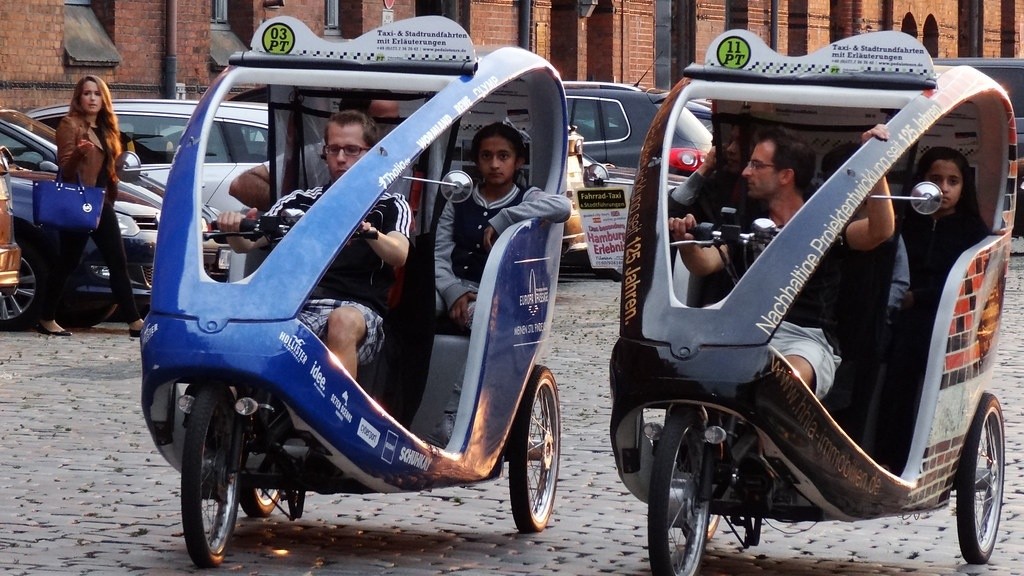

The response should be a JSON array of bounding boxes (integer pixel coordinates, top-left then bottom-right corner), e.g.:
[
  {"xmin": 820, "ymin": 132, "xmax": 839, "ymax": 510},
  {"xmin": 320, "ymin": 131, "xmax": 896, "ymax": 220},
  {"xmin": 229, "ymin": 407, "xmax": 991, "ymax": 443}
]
[{"xmin": 31, "ymin": 156, "xmax": 108, "ymax": 234}]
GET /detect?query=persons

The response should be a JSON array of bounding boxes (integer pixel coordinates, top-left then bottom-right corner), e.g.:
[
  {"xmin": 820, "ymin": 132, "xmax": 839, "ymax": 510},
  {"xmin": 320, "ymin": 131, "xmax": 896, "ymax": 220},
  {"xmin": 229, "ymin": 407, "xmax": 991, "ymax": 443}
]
[
  {"xmin": 218, "ymin": 110, "xmax": 413, "ymax": 381},
  {"xmin": 425, "ymin": 122, "xmax": 573, "ymax": 449},
  {"xmin": 668, "ymin": 114, "xmax": 910, "ymax": 440},
  {"xmin": 878, "ymin": 147, "xmax": 1005, "ymax": 472},
  {"xmin": 229, "ymin": 88, "xmax": 418, "ymax": 213},
  {"xmin": 35, "ymin": 76, "xmax": 144, "ymax": 337}
]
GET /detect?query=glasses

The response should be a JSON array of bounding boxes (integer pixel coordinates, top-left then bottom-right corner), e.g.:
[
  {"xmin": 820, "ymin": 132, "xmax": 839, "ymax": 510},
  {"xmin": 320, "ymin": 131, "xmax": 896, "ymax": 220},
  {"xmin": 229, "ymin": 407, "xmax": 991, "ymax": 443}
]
[
  {"xmin": 747, "ymin": 160, "xmax": 776, "ymax": 171},
  {"xmin": 322, "ymin": 143, "xmax": 372, "ymax": 157}
]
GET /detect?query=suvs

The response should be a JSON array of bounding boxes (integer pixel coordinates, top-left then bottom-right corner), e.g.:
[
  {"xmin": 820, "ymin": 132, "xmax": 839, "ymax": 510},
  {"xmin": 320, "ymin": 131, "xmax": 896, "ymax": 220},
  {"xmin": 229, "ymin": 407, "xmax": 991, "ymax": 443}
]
[
  {"xmin": 20, "ymin": 95, "xmax": 329, "ymax": 222},
  {"xmin": 545, "ymin": 79, "xmax": 725, "ymax": 281}
]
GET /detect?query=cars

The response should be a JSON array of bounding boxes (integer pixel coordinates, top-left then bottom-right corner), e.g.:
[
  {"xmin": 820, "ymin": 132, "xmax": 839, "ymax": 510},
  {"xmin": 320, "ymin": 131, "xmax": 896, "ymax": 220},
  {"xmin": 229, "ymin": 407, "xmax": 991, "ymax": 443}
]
[
  {"xmin": 0, "ymin": 104, "xmax": 244, "ymax": 331},
  {"xmin": 923, "ymin": 54, "xmax": 1024, "ymax": 224}
]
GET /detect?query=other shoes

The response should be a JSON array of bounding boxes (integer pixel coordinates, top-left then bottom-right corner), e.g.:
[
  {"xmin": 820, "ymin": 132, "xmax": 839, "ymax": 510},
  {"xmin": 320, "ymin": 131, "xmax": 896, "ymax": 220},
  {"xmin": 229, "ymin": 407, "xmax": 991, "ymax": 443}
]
[
  {"xmin": 128, "ymin": 328, "xmax": 141, "ymax": 337},
  {"xmin": 34, "ymin": 320, "xmax": 74, "ymax": 337}
]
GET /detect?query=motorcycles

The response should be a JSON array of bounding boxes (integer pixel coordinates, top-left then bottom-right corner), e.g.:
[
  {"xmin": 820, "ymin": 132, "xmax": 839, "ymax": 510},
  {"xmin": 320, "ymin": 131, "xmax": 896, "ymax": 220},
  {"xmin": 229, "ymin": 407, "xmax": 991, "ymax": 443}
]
[
  {"xmin": 605, "ymin": 29, "xmax": 1018, "ymax": 576},
  {"xmin": 141, "ymin": 14, "xmax": 571, "ymax": 566}
]
